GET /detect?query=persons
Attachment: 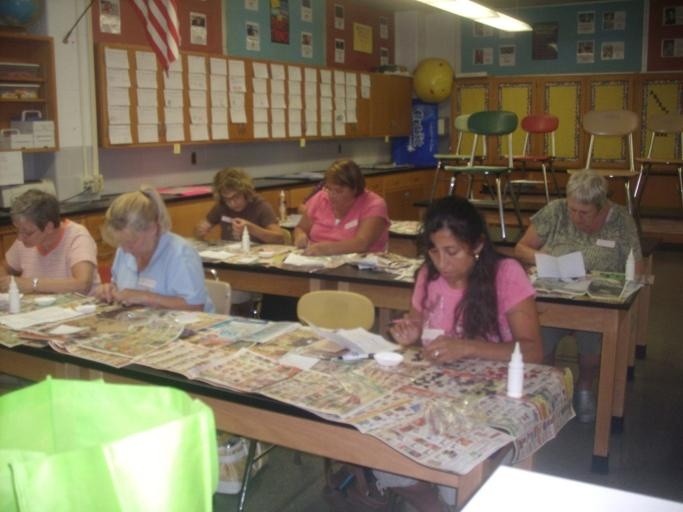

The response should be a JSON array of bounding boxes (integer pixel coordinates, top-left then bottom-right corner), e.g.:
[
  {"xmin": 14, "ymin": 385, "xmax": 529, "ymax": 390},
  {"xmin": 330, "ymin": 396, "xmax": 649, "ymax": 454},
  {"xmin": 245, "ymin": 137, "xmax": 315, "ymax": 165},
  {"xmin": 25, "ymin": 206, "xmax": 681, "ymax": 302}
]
[
  {"xmin": 258, "ymin": 157, "xmax": 391, "ymax": 326},
  {"xmin": 94, "ymin": 182, "xmax": 217, "ymax": 315},
  {"xmin": 0, "ymin": 187, "xmax": 101, "ymax": 302},
  {"xmin": 194, "ymin": 168, "xmax": 285, "ymax": 317},
  {"xmin": 513, "ymin": 169, "xmax": 645, "ymax": 427},
  {"xmin": 369, "ymin": 194, "xmax": 545, "ymax": 512}
]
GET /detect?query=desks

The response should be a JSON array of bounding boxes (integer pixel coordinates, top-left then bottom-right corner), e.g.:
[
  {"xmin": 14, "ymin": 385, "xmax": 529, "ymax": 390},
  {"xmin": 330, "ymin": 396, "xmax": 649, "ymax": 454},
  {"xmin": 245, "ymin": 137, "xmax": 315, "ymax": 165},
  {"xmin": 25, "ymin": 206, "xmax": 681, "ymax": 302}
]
[{"xmin": 0, "ymin": 185, "xmax": 683, "ymax": 512}]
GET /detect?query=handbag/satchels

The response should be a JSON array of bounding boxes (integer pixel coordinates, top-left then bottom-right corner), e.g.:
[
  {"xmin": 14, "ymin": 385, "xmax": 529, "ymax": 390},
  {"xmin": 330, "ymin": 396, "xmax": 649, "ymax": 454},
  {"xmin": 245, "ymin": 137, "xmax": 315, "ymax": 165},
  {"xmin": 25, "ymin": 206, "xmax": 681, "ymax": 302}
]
[{"xmin": 217, "ymin": 438, "xmax": 262, "ymax": 494}]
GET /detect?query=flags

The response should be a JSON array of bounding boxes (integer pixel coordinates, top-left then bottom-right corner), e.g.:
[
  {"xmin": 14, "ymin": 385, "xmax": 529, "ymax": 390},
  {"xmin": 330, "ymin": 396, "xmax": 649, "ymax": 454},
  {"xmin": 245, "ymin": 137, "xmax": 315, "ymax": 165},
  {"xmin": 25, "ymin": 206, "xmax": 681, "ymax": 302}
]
[{"xmin": 131, "ymin": 0, "xmax": 182, "ymax": 77}]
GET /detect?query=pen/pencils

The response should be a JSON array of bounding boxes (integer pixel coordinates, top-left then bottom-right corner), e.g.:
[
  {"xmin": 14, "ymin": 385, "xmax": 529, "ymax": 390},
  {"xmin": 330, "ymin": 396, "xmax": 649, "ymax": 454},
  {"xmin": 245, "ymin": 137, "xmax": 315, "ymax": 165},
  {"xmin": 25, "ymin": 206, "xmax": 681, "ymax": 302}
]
[
  {"xmin": 390, "ymin": 318, "xmax": 419, "ymax": 323},
  {"xmin": 339, "ymin": 353, "xmax": 374, "ymax": 360}
]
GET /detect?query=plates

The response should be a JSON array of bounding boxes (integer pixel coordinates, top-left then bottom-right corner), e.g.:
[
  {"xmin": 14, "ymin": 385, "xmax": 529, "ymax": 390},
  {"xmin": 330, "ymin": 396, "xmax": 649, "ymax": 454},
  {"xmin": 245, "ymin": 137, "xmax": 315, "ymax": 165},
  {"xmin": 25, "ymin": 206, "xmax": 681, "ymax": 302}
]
[
  {"xmin": 375, "ymin": 353, "xmax": 403, "ymax": 368},
  {"xmin": 76, "ymin": 304, "xmax": 95, "ymax": 314},
  {"xmin": 34, "ymin": 297, "xmax": 56, "ymax": 306}
]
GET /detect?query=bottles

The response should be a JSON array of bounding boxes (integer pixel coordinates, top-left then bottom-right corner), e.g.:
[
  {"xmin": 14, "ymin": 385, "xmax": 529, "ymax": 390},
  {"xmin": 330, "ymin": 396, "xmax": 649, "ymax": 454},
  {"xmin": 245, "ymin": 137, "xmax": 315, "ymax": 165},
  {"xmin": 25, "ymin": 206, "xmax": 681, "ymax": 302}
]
[
  {"xmin": 9, "ymin": 275, "xmax": 20, "ymax": 312},
  {"xmin": 506, "ymin": 342, "xmax": 526, "ymax": 398},
  {"xmin": 242, "ymin": 225, "xmax": 251, "ymax": 253},
  {"xmin": 624, "ymin": 248, "xmax": 636, "ymax": 281},
  {"xmin": 279, "ymin": 190, "xmax": 287, "ymax": 220}
]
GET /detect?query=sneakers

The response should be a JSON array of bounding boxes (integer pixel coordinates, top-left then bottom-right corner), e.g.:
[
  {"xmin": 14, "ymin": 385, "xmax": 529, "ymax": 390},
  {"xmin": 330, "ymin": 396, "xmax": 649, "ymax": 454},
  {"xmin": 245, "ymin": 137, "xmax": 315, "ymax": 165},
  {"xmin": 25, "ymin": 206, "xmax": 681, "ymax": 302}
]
[{"xmin": 573, "ymin": 390, "xmax": 597, "ymax": 423}]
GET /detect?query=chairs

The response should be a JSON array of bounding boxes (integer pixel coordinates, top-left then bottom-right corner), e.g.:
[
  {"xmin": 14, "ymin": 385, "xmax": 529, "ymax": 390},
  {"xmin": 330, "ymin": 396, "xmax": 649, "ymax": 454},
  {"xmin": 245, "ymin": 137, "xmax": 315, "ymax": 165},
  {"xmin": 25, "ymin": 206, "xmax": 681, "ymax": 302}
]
[{"xmin": 430, "ymin": 110, "xmax": 683, "ymax": 239}]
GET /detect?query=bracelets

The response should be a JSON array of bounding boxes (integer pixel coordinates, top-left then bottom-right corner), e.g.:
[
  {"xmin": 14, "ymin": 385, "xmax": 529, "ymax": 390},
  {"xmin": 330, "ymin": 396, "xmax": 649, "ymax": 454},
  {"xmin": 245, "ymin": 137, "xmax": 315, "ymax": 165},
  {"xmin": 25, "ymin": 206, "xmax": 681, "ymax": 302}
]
[{"xmin": 32, "ymin": 277, "xmax": 38, "ymax": 292}]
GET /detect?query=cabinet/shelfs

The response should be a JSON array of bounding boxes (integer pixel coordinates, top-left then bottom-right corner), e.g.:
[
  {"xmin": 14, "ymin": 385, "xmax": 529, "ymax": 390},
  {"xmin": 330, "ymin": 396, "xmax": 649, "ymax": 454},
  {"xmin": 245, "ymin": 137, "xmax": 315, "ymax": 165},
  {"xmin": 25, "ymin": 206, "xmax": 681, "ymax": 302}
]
[{"xmin": 0, "ymin": 32, "xmax": 59, "ymax": 153}]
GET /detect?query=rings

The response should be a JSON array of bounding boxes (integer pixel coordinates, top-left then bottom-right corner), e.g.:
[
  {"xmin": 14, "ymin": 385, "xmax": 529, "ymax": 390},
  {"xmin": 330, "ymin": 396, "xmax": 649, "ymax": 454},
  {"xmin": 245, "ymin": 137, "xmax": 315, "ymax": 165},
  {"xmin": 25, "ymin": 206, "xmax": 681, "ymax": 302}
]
[{"xmin": 435, "ymin": 350, "xmax": 440, "ymax": 359}]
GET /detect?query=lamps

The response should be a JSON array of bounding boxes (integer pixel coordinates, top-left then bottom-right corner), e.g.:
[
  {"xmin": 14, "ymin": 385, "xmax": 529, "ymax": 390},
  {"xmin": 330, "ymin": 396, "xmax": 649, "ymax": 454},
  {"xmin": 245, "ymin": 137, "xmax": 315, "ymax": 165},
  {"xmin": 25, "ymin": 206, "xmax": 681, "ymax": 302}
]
[{"xmin": 414, "ymin": 0, "xmax": 534, "ymax": 33}]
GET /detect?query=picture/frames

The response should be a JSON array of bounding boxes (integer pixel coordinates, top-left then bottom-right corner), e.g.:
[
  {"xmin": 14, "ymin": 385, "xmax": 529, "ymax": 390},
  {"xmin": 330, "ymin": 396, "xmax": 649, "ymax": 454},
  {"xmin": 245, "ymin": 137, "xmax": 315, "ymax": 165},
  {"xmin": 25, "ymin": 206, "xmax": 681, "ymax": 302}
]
[{"xmin": 454, "ymin": 79, "xmax": 683, "ymax": 164}]
